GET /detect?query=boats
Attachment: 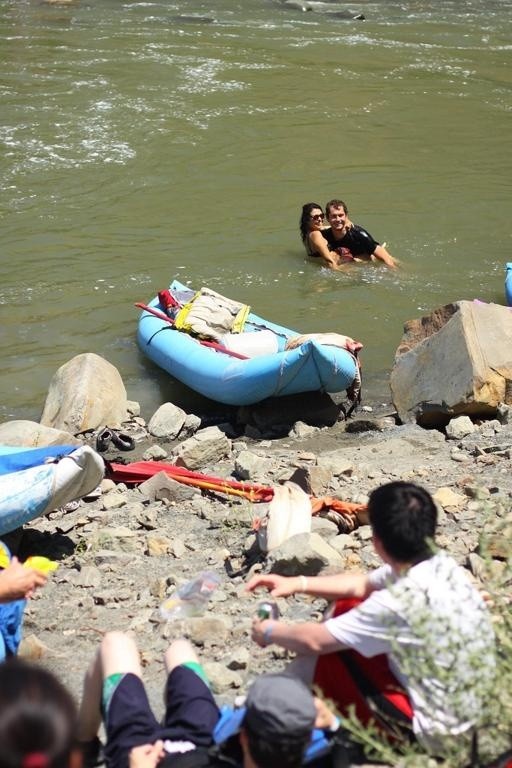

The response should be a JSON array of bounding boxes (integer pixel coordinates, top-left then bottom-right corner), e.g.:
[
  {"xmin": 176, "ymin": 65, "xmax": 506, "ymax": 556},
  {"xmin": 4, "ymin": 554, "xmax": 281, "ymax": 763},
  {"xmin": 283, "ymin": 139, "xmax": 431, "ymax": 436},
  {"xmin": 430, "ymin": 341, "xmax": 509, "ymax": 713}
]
[
  {"xmin": 1, "ymin": 440, "xmax": 109, "ymax": 540},
  {"xmin": 133, "ymin": 278, "xmax": 364, "ymax": 411}
]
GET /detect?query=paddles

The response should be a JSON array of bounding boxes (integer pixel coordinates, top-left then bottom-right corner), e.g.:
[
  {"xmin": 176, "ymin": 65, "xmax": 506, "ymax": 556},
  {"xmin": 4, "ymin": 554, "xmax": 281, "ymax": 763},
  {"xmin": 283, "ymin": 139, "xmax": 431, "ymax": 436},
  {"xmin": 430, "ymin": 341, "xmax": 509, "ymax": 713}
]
[
  {"xmin": 109, "ymin": 461, "xmax": 275, "ymax": 494},
  {"xmin": 108, "ymin": 473, "xmax": 368, "ymax": 513}
]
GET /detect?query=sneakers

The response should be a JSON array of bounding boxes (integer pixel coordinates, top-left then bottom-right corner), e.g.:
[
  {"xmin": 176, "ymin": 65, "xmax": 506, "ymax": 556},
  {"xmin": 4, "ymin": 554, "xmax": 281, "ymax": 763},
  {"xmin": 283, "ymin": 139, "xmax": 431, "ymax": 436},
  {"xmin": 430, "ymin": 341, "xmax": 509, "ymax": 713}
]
[
  {"xmin": 113, "ymin": 432, "xmax": 135, "ymax": 450},
  {"xmin": 97, "ymin": 428, "xmax": 113, "ymax": 451}
]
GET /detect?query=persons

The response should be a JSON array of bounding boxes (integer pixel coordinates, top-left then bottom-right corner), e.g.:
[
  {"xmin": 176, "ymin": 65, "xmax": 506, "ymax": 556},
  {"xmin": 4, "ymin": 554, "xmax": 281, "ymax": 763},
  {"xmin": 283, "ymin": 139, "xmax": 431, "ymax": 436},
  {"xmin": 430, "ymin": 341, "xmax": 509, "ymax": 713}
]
[
  {"xmin": 299, "ymin": 203, "xmax": 375, "ymax": 272},
  {"xmin": 75, "ymin": 631, "xmax": 367, "ymax": 767},
  {"xmin": 0, "ymin": 539, "xmax": 49, "ymax": 659},
  {"xmin": 0, "ymin": 653, "xmax": 85, "ymax": 768},
  {"xmin": 321, "ymin": 199, "xmax": 398, "ymax": 272},
  {"xmin": 244, "ymin": 482, "xmax": 496, "ymax": 763}
]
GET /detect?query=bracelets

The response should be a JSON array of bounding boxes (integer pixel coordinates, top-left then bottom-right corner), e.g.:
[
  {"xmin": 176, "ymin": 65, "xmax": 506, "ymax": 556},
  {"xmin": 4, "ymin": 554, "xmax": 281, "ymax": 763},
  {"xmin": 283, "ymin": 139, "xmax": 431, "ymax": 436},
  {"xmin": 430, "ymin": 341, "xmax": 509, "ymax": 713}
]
[
  {"xmin": 298, "ymin": 572, "xmax": 310, "ymax": 594},
  {"xmin": 262, "ymin": 622, "xmax": 275, "ymax": 646},
  {"xmin": 330, "ymin": 716, "xmax": 342, "ymax": 733}
]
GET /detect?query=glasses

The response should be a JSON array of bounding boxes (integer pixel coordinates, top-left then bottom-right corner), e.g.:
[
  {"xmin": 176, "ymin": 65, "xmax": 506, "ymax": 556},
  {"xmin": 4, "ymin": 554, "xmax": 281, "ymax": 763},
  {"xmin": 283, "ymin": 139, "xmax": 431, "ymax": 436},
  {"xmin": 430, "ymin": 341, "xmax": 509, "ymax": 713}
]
[{"xmin": 307, "ymin": 214, "xmax": 324, "ymax": 220}]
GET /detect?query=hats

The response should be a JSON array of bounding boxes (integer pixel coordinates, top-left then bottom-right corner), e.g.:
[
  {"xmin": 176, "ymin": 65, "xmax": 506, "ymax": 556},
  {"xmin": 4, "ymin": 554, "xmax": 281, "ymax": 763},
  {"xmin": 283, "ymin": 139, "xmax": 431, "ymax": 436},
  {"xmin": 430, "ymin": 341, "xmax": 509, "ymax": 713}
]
[{"xmin": 241, "ymin": 676, "xmax": 317, "ymax": 742}]
